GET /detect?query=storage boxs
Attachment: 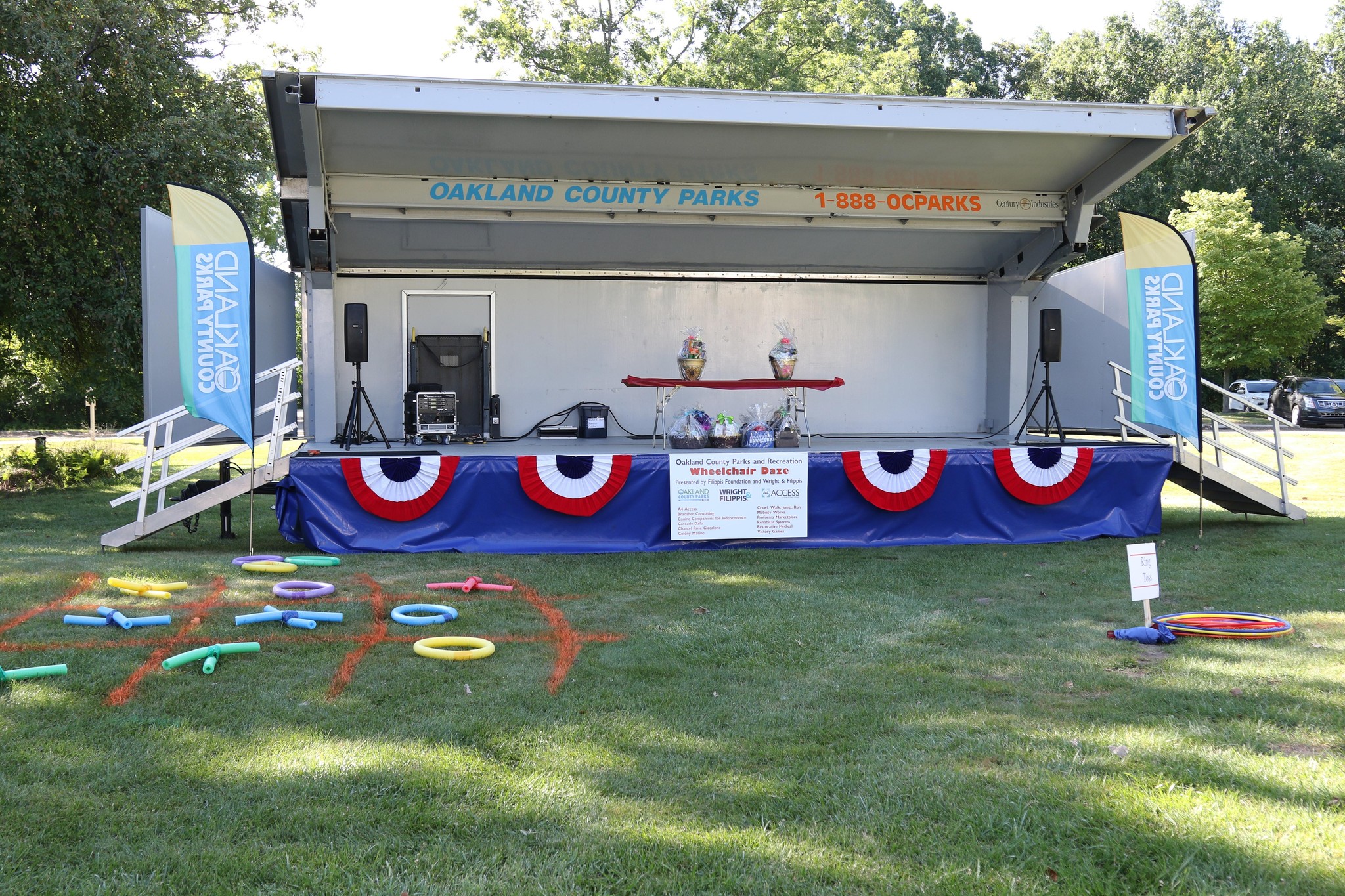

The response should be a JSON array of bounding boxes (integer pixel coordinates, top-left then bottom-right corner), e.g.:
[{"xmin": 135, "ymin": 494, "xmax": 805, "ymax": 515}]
[
  {"xmin": 774, "ymin": 431, "xmax": 800, "ymax": 447},
  {"xmin": 741, "ymin": 430, "xmax": 774, "ymax": 448},
  {"xmin": 577, "ymin": 406, "xmax": 610, "ymax": 439}
]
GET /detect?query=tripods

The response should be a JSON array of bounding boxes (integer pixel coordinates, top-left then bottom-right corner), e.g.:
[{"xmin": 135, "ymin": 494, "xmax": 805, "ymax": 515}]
[
  {"xmin": 339, "ymin": 362, "xmax": 391, "ymax": 451},
  {"xmin": 1014, "ymin": 362, "xmax": 1065, "ymax": 442}
]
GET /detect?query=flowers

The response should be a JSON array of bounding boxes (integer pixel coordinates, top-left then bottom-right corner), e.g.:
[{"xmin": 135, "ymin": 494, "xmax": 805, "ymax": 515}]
[{"xmin": 781, "ymin": 338, "xmax": 791, "ymax": 344}]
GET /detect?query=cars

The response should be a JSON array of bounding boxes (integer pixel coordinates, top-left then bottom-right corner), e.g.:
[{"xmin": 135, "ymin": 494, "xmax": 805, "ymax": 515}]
[
  {"xmin": 1228, "ymin": 379, "xmax": 1284, "ymax": 412},
  {"xmin": 1332, "ymin": 379, "xmax": 1345, "ymax": 391},
  {"xmin": 1269, "ymin": 376, "xmax": 1345, "ymax": 428}
]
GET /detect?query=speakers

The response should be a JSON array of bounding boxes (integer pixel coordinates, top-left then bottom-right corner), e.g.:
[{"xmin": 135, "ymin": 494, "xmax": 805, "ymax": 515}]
[
  {"xmin": 414, "ymin": 335, "xmax": 484, "ymax": 437},
  {"xmin": 1039, "ymin": 309, "xmax": 1062, "ymax": 362},
  {"xmin": 344, "ymin": 303, "xmax": 368, "ymax": 362}
]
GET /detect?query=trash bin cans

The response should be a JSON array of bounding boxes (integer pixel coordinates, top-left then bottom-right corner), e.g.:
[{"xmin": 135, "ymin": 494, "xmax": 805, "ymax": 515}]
[{"xmin": 34, "ymin": 436, "xmax": 46, "ymax": 452}]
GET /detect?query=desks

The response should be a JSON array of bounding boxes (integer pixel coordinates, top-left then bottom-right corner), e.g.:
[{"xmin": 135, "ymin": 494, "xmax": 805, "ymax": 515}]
[{"xmin": 621, "ymin": 375, "xmax": 845, "ymax": 450}]
